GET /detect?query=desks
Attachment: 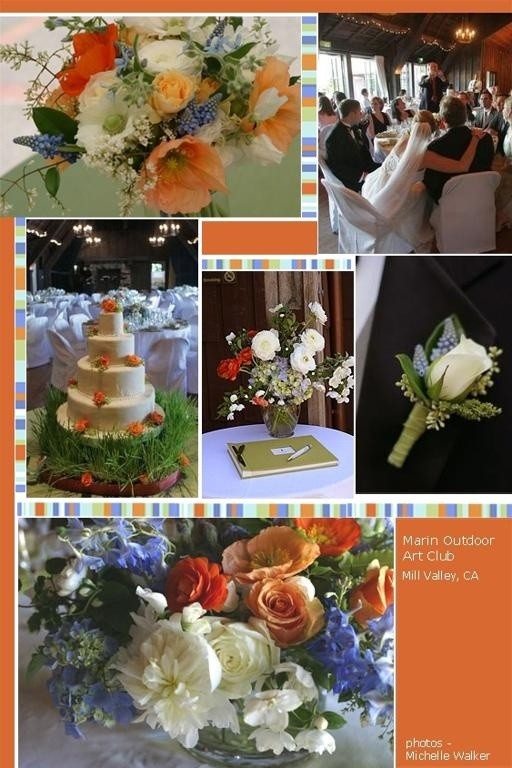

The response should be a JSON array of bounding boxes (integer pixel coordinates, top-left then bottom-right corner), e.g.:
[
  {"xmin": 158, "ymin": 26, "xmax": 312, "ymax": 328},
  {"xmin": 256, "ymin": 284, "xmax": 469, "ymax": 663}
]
[{"xmin": 203, "ymin": 417, "xmax": 356, "ymax": 494}]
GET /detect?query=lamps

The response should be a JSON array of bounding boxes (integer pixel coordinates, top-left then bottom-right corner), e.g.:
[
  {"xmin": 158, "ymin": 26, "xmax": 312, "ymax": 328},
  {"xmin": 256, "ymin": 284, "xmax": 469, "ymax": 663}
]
[{"xmin": 453, "ymin": 20, "xmax": 477, "ymax": 46}]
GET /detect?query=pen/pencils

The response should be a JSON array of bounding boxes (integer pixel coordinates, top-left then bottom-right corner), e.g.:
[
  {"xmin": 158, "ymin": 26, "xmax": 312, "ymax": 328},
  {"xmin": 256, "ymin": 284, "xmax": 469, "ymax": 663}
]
[
  {"xmin": 238, "ymin": 444, "xmax": 245, "ymax": 454},
  {"xmin": 288, "ymin": 444, "xmax": 313, "ymax": 462}
]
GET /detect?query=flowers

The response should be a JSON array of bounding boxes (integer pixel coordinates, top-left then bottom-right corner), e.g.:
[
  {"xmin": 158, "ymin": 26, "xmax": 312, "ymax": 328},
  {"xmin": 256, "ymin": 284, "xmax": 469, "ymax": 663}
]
[
  {"xmin": 212, "ymin": 290, "xmax": 355, "ymax": 430},
  {"xmin": 0, "ymin": 18, "xmax": 300, "ymax": 220},
  {"xmin": 14, "ymin": 517, "xmax": 395, "ymax": 766},
  {"xmin": 381, "ymin": 305, "xmax": 506, "ymax": 471}
]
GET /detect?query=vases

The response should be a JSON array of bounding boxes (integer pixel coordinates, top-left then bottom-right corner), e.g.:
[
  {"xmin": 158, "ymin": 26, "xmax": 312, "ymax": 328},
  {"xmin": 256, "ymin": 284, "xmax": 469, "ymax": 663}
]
[
  {"xmin": 156, "ymin": 670, "xmax": 325, "ymax": 763},
  {"xmin": 257, "ymin": 393, "xmax": 301, "ymax": 438},
  {"xmin": 146, "ymin": 162, "xmax": 232, "ymax": 219}
]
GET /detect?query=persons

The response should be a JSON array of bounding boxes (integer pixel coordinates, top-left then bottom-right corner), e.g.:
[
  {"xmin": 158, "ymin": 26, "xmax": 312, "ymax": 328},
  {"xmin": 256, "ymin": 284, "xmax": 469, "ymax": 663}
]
[{"xmin": 318, "ymin": 63, "xmax": 512, "ymax": 219}]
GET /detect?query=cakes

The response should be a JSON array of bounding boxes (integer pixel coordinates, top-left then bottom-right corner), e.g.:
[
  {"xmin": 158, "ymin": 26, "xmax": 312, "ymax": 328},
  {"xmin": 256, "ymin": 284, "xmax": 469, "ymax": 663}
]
[{"xmin": 67, "ymin": 308, "xmax": 155, "ymax": 430}]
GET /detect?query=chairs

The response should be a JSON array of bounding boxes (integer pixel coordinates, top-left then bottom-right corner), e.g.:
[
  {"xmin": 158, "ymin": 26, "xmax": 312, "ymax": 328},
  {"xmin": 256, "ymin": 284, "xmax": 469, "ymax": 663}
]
[
  {"xmin": 25, "ymin": 276, "xmax": 197, "ymax": 497},
  {"xmin": 319, "ymin": 77, "xmax": 512, "ymax": 254}
]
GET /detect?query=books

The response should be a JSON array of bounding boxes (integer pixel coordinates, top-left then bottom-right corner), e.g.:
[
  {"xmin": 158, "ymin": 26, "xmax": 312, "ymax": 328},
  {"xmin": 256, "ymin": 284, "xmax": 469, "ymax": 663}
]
[{"xmin": 225, "ymin": 435, "xmax": 339, "ymax": 479}]
